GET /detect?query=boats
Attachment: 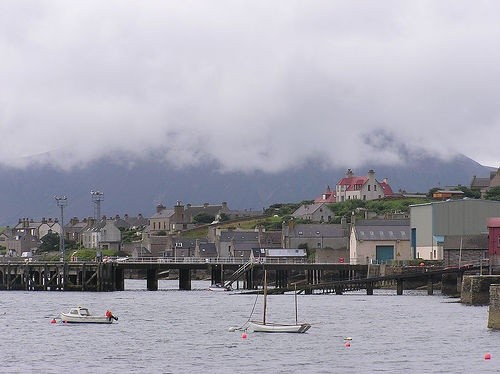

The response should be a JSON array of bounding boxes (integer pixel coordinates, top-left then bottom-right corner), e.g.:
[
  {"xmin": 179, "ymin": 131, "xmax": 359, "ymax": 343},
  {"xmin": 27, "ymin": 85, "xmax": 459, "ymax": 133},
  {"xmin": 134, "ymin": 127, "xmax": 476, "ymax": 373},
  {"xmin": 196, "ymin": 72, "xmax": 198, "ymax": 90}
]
[{"xmin": 49, "ymin": 306, "xmax": 119, "ymax": 324}]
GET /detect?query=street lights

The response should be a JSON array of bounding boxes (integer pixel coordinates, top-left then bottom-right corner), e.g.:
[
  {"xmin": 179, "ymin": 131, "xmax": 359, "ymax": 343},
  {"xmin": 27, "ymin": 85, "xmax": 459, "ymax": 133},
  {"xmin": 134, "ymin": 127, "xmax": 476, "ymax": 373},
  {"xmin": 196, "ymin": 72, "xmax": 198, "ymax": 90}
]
[
  {"xmin": 89, "ymin": 190, "xmax": 106, "ymax": 257},
  {"xmin": 53, "ymin": 195, "xmax": 68, "ymax": 259}
]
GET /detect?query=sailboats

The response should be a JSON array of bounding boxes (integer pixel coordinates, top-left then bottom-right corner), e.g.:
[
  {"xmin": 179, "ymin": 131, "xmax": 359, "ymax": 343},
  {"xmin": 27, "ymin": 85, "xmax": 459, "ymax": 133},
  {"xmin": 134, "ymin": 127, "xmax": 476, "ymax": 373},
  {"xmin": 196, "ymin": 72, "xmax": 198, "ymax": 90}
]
[{"xmin": 228, "ymin": 270, "xmax": 312, "ymax": 334}]
